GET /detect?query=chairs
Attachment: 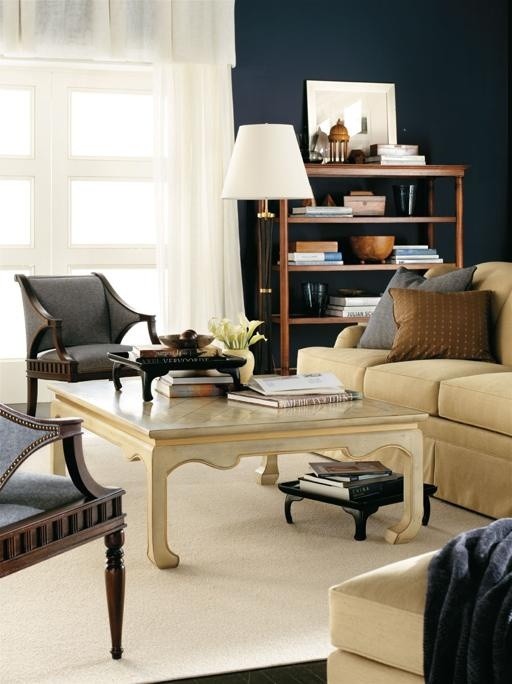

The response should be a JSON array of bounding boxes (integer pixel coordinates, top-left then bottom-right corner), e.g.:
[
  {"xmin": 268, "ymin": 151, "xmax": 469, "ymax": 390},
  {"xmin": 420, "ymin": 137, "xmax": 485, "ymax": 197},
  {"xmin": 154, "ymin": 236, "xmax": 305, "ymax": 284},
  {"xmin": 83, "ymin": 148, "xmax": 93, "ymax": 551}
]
[
  {"xmin": 12, "ymin": 272, "xmax": 161, "ymax": 417},
  {"xmin": 1, "ymin": 402, "xmax": 126, "ymax": 660}
]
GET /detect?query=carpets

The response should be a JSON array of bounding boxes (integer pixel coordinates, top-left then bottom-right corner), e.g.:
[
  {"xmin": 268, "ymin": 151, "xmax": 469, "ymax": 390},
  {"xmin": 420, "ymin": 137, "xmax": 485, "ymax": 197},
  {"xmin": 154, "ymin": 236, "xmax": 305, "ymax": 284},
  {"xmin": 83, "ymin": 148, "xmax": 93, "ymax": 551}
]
[{"xmin": 0, "ymin": 418, "xmax": 498, "ymax": 684}]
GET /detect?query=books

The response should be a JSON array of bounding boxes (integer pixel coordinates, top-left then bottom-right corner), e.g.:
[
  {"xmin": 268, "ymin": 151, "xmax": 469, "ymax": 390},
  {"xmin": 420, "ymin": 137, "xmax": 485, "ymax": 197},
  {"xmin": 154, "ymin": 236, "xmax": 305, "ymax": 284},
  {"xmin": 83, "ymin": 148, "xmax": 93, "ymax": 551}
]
[
  {"xmin": 364, "ymin": 154, "xmax": 425, "ymax": 165},
  {"xmin": 128, "ymin": 345, "xmax": 226, "ymax": 364},
  {"xmin": 294, "ymin": 460, "xmax": 404, "ymax": 505},
  {"xmin": 287, "ymin": 205, "xmax": 356, "ymax": 220},
  {"xmin": 152, "ymin": 369, "xmax": 235, "ymax": 400},
  {"xmin": 324, "ymin": 290, "xmax": 383, "ymax": 319},
  {"xmin": 386, "ymin": 245, "xmax": 445, "ymax": 266},
  {"xmin": 275, "ymin": 239, "xmax": 344, "ymax": 267},
  {"xmin": 226, "ymin": 371, "xmax": 363, "ymax": 410}
]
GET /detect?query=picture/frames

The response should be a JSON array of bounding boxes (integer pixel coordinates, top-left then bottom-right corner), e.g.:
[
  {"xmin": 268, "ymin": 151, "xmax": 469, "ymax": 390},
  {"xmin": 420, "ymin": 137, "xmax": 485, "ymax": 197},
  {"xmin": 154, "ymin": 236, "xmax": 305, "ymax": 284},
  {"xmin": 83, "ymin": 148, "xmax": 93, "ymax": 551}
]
[{"xmin": 304, "ymin": 79, "xmax": 399, "ymax": 163}]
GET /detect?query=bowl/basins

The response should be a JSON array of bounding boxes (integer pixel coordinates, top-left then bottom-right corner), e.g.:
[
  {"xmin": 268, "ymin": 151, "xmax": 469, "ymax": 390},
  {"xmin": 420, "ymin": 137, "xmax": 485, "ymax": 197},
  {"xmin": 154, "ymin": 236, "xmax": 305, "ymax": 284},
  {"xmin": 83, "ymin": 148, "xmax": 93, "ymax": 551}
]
[{"xmin": 350, "ymin": 235, "xmax": 395, "ymax": 259}]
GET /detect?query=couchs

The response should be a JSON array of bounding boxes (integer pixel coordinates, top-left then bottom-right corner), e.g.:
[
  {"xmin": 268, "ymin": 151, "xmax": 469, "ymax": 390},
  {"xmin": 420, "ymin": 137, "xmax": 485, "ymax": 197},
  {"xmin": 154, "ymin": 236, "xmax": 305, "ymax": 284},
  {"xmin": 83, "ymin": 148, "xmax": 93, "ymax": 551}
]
[
  {"xmin": 297, "ymin": 260, "xmax": 512, "ymax": 521},
  {"xmin": 326, "ymin": 547, "xmax": 441, "ymax": 684}
]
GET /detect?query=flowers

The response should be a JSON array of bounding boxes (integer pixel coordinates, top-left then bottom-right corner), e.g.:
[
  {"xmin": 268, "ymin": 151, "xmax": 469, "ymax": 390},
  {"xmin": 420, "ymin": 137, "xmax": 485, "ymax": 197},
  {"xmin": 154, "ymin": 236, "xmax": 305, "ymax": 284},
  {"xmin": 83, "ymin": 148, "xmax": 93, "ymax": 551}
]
[{"xmin": 209, "ymin": 312, "xmax": 267, "ymax": 350}]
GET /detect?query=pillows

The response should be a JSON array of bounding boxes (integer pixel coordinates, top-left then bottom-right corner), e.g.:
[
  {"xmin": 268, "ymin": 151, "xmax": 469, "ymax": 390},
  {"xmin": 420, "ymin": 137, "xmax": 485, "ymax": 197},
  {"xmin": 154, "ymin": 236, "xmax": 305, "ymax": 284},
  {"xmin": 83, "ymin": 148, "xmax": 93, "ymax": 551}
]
[
  {"xmin": 385, "ymin": 287, "xmax": 498, "ymax": 364},
  {"xmin": 356, "ymin": 266, "xmax": 479, "ymax": 349}
]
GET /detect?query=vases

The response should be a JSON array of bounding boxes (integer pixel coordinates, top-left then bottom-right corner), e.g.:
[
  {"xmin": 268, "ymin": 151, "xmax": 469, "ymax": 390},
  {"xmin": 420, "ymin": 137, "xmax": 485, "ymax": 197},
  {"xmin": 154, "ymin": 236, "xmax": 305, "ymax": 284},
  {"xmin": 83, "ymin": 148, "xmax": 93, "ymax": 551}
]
[
  {"xmin": 394, "ymin": 185, "xmax": 416, "ymax": 216},
  {"xmin": 221, "ymin": 351, "xmax": 254, "ymax": 385}
]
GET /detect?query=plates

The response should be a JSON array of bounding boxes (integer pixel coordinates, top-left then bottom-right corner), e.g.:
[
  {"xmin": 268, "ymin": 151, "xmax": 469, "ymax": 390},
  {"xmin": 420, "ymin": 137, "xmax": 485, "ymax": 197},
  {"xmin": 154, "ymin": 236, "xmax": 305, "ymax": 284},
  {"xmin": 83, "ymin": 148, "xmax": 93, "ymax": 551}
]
[{"xmin": 158, "ymin": 334, "xmax": 216, "ymax": 349}]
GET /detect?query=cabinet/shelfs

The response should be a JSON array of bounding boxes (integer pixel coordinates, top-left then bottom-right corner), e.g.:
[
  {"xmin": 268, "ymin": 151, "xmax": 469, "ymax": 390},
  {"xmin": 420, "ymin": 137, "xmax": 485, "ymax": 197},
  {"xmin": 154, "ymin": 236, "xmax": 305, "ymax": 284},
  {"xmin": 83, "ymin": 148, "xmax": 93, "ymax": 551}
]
[{"xmin": 255, "ymin": 164, "xmax": 466, "ymax": 375}]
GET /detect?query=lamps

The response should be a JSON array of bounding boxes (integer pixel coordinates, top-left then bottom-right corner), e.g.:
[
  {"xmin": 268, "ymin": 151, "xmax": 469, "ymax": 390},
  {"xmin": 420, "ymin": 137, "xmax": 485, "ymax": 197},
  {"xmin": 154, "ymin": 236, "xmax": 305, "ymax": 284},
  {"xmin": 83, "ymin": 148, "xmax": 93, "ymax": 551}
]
[{"xmin": 220, "ymin": 121, "xmax": 315, "ymax": 374}]
